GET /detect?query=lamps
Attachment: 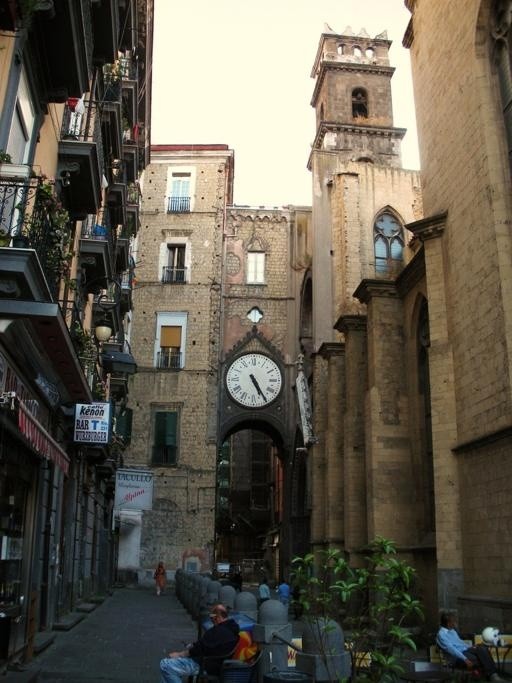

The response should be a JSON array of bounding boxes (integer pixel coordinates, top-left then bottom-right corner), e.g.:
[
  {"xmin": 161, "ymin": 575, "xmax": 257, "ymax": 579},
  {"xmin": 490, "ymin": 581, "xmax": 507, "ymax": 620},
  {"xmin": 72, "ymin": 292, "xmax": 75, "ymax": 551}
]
[{"xmin": 77, "ymin": 276, "xmax": 123, "ymax": 343}]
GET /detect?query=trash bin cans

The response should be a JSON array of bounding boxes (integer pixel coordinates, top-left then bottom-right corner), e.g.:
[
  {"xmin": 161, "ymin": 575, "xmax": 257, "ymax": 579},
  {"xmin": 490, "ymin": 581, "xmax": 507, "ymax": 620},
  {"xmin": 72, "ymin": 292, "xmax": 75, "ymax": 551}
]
[{"xmin": 263, "ymin": 670, "xmax": 312, "ymax": 683}]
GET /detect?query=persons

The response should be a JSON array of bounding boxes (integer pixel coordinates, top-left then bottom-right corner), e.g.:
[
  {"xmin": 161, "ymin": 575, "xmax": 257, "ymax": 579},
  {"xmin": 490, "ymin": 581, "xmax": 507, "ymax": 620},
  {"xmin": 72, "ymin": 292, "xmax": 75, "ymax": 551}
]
[
  {"xmin": 435, "ymin": 607, "xmax": 507, "ymax": 683},
  {"xmin": 258, "ymin": 576, "xmax": 271, "ymax": 604},
  {"xmin": 154, "ymin": 561, "xmax": 168, "ymax": 597},
  {"xmin": 234, "ymin": 570, "xmax": 244, "ymax": 592},
  {"xmin": 157, "ymin": 603, "xmax": 241, "ymax": 683},
  {"xmin": 276, "ymin": 579, "xmax": 291, "ymax": 606}
]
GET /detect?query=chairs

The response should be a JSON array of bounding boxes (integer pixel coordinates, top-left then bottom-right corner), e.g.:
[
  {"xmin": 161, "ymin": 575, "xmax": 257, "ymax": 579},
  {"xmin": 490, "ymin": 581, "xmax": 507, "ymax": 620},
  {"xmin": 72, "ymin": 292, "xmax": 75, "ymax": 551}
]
[
  {"xmin": 427, "ymin": 632, "xmax": 511, "ymax": 683},
  {"xmin": 198, "ymin": 631, "xmax": 265, "ymax": 683}
]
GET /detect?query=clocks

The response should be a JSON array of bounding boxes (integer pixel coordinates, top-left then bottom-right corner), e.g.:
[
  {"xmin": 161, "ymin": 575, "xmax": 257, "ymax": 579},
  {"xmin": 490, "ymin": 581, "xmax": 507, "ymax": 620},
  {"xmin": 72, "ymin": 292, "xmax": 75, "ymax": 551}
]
[{"xmin": 227, "ymin": 351, "xmax": 284, "ymax": 410}]
